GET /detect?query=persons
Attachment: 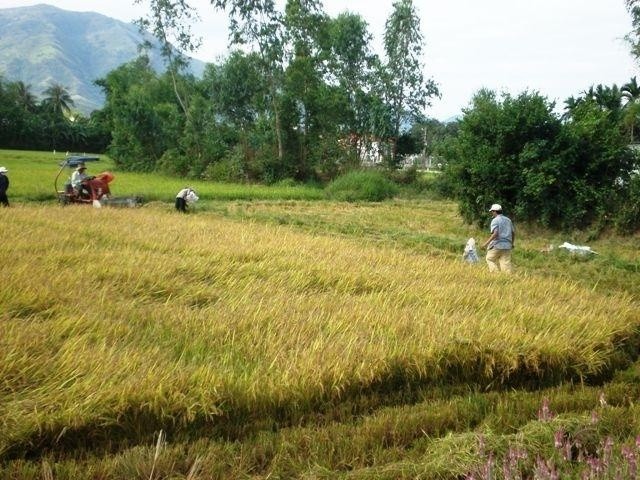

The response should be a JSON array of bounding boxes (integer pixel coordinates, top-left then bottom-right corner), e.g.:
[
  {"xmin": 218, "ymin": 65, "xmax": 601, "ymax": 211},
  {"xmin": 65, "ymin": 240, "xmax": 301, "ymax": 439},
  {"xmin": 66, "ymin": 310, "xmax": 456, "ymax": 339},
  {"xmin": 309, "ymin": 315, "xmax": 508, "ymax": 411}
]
[
  {"xmin": 0, "ymin": 167, "xmax": 11, "ymax": 208},
  {"xmin": 71, "ymin": 163, "xmax": 97, "ymax": 199},
  {"xmin": 480, "ymin": 203, "xmax": 515, "ymax": 274},
  {"xmin": 175, "ymin": 186, "xmax": 199, "ymax": 214}
]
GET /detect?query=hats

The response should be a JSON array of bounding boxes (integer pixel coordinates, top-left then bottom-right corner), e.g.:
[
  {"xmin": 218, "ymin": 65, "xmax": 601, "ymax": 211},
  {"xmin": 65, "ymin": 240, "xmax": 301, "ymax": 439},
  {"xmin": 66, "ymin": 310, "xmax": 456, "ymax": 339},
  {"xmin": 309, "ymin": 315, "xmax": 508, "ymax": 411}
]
[
  {"xmin": 489, "ymin": 203, "xmax": 502, "ymax": 212},
  {"xmin": 76, "ymin": 164, "xmax": 87, "ymax": 171},
  {"xmin": 0, "ymin": 167, "xmax": 9, "ymax": 173}
]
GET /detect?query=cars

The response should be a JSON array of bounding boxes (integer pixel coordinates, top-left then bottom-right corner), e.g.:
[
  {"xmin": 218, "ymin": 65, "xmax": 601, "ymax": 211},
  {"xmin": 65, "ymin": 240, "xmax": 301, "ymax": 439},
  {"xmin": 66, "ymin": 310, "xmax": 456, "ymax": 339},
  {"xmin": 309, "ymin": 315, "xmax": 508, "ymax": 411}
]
[{"xmin": 60, "ymin": 156, "xmax": 84, "ymax": 167}]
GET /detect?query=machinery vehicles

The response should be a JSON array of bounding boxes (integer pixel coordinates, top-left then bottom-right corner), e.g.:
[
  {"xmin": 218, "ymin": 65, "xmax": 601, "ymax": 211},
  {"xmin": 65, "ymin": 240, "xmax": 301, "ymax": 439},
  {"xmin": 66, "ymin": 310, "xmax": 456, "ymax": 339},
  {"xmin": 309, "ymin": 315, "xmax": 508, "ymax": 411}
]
[{"xmin": 55, "ymin": 160, "xmax": 116, "ymax": 207}]
